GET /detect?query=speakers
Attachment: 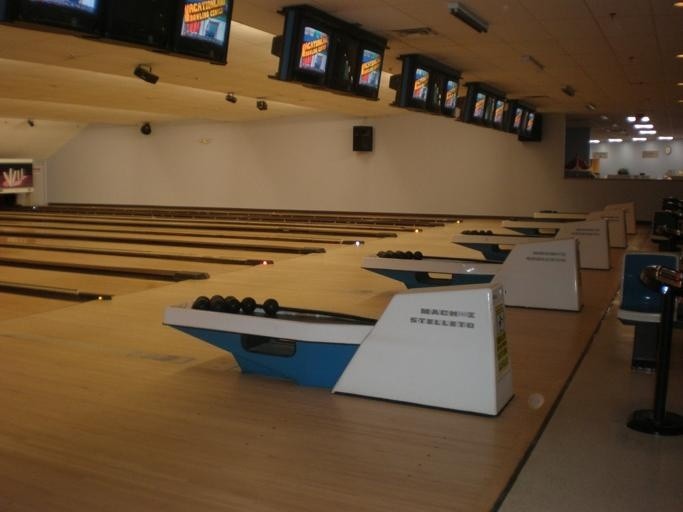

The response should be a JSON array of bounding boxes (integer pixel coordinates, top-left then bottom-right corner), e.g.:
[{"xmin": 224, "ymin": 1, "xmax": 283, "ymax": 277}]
[{"xmin": 353, "ymin": 126, "xmax": 372, "ymax": 151}]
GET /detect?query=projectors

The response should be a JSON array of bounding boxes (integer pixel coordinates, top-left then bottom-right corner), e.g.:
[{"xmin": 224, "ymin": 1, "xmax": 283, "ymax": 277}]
[
  {"xmin": 226, "ymin": 95, "xmax": 236, "ymax": 103},
  {"xmin": 134, "ymin": 67, "xmax": 159, "ymax": 83}
]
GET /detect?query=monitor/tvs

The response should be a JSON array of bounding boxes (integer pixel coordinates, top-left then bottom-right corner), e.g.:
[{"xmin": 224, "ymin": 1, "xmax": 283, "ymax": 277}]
[
  {"xmin": 351, "ymin": 41, "xmax": 384, "ymax": 99},
  {"xmin": 441, "ymin": 76, "xmax": 459, "ymax": 116},
  {"xmin": 278, "ymin": 8, "xmax": 340, "ymax": 90},
  {"xmin": 463, "ymin": 87, "xmax": 538, "ymax": 137},
  {"xmin": 166, "ymin": 0, "xmax": 234, "ymax": 63},
  {"xmin": 399, "ymin": 59, "xmax": 436, "ymax": 112},
  {"xmin": 0, "ymin": 0, "xmax": 110, "ymax": 38}
]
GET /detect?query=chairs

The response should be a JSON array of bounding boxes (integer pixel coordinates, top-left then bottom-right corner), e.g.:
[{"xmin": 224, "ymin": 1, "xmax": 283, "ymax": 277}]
[{"xmin": 616, "ymin": 196, "xmax": 682, "ymax": 375}]
